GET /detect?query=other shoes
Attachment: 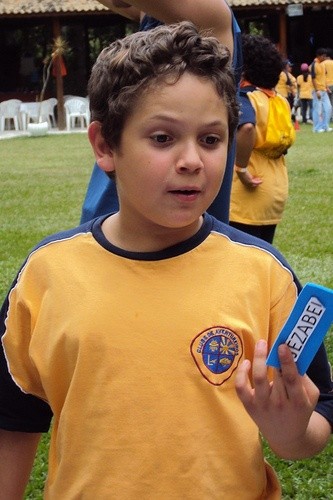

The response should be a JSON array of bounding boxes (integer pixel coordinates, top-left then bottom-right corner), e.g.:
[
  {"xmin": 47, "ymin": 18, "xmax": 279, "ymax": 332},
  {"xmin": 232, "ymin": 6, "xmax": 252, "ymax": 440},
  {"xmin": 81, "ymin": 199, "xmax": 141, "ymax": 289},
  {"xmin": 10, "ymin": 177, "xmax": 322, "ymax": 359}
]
[
  {"xmin": 307, "ymin": 119, "xmax": 313, "ymax": 125},
  {"xmin": 325, "ymin": 127, "xmax": 333, "ymax": 132},
  {"xmin": 313, "ymin": 128, "xmax": 325, "ymax": 133}
]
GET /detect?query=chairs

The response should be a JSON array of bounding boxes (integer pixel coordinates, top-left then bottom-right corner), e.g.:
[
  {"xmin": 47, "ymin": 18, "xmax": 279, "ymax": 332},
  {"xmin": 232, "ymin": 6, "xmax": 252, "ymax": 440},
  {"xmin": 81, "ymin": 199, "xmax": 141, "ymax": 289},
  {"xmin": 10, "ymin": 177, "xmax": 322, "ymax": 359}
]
[{"xmin": 0, "ymin": 96, "xmax": 89, "ymax": 134}]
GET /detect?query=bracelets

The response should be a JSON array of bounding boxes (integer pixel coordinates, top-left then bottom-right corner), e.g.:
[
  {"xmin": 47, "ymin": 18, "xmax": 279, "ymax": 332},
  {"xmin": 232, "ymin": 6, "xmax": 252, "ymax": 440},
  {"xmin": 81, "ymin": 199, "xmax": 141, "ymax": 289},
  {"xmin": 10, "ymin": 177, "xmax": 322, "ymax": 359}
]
[{"xmin": 234, "ymin": 164, "xmax": 247, "ymax": 174}]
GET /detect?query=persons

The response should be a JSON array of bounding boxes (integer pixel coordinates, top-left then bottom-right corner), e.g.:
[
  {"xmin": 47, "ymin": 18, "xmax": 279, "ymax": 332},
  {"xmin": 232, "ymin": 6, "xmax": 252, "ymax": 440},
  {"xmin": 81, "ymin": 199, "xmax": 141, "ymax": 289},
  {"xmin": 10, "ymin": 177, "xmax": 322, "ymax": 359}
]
[
  {"xmin": 275, "ymin": 47, "xmax": 333, "ymax": 134},
  {"xmin": 228, "ymin": 32, "xmax": 296, "ymax": 246},
  {"xmin": 0, "ymin": 22, "xmax": 333, "ymax": 500},
  {"xmin": 80, "ymin": 0, "xmax": 242, "ymax": 228}
]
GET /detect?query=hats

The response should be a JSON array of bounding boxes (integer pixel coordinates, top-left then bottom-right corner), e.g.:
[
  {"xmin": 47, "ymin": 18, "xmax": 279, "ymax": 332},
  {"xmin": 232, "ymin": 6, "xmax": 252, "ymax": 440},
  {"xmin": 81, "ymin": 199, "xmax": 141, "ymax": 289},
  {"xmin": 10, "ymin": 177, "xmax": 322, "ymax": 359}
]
[
  {"xmin": 283, "ymin": 59, "xmax": 294, "ymax": 67},
  {"xmin": 301, "ymin": 63, "xmax": 308, "ymax": 70}
]
